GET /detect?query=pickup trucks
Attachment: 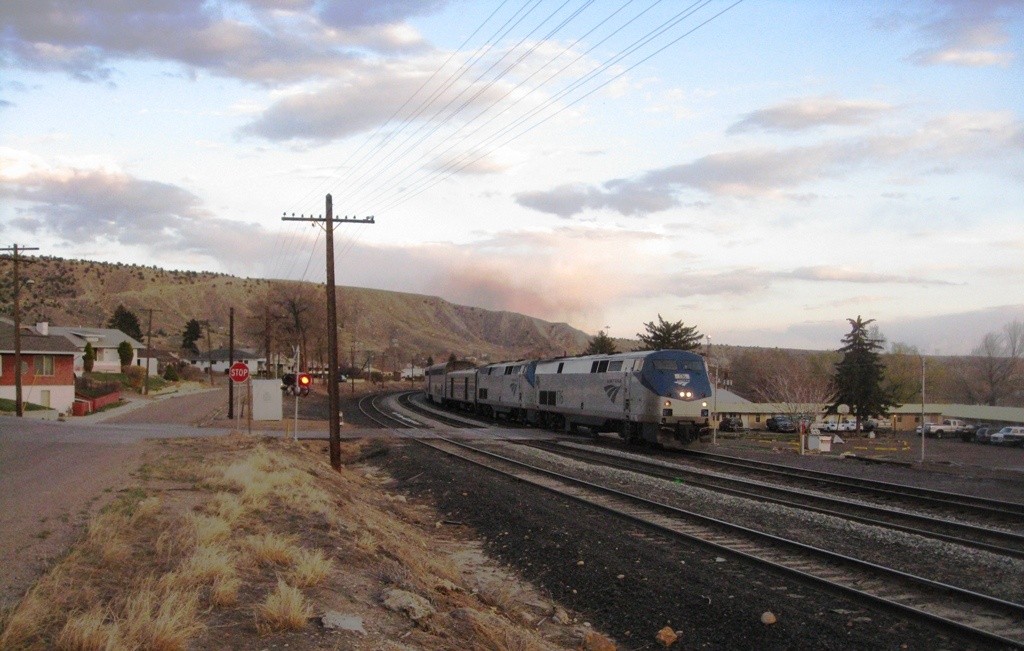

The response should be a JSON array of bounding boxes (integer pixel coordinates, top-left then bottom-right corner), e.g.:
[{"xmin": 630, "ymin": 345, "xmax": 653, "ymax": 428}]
[
  {"xmin": 926, "ymin": 418, "xmax": 967, "ymax": 439},
  {"xmin": 956, "ymin": 423, "xmax": 993, "ymax": 443}
]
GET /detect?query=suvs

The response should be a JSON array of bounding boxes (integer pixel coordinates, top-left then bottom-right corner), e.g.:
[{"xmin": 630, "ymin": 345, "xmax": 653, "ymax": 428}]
[{"xmin": 975, "ymin": 423, "xmax": 1024, "ymax": 448}]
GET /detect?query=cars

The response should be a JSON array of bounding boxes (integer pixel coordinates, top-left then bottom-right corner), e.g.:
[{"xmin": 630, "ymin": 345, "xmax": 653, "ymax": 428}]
[
  {"xmin": 719, "ymin": 416, "xmax": 744, "ymax": 432},
  {"xmin": 915, "ymin": 422, "xmax": 939, "ymax": 437},
  {"xmin": 765, "ymin": 416, "xmax": 795, "ymax": 434},
  {"xmin": 862, "ymin": 416, "xmax": 892, "ymax": 433},
  {"xmin": 822, "ymin": 418, "xmax": 846, "ymax": 433},
  {"xmin": 841, "ymin": 419, "xmax": 864, "ymax": 433}
]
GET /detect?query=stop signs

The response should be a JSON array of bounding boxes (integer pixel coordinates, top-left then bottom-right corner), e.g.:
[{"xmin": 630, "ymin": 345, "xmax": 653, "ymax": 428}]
[{"xmin": 229, "ymin": 362, "xmax": 249, "ymax": 383}]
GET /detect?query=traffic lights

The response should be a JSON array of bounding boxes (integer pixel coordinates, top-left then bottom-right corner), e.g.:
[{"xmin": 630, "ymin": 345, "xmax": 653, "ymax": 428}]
[{"xmin": 298, "ymin": 373, "xmax": 312, "ymax": 387}]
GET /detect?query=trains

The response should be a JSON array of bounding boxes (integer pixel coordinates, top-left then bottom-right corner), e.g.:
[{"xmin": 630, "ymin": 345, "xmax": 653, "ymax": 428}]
[{"xmin": 423, "ymin": 346, "xmax": 718, "ymax": 449}]
